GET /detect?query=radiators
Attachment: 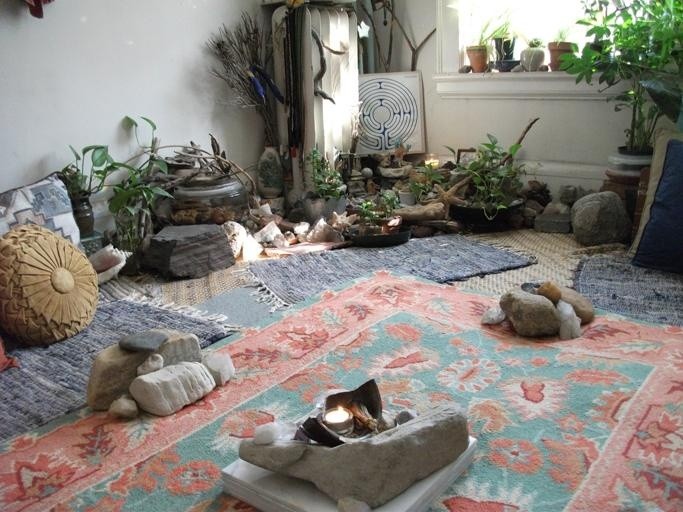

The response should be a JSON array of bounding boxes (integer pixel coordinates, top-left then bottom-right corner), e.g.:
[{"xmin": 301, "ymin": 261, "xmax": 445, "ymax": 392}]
[{"xmin": 268, "ymin": 9, "xmax": 363, "ymax": 204}]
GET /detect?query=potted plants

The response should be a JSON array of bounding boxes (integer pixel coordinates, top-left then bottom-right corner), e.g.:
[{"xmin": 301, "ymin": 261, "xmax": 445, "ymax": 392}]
[
  {"xmin": 103, "ymin": 112, "xmax": 168, "ymax": 278},
  {"xmin": 60, "ymin": 142, "xmax": 118, "ymax": 237},
  {"xmin": 463, "ymin": 23, "xmax": 612, "ymax": 74},
  {"xmin": 557, "ymin": 0, "xmax": 683, "ymax": 171},
  {"xmin": 293, "ymin": 144, "xmax": 532, "ymax": 249}
]
